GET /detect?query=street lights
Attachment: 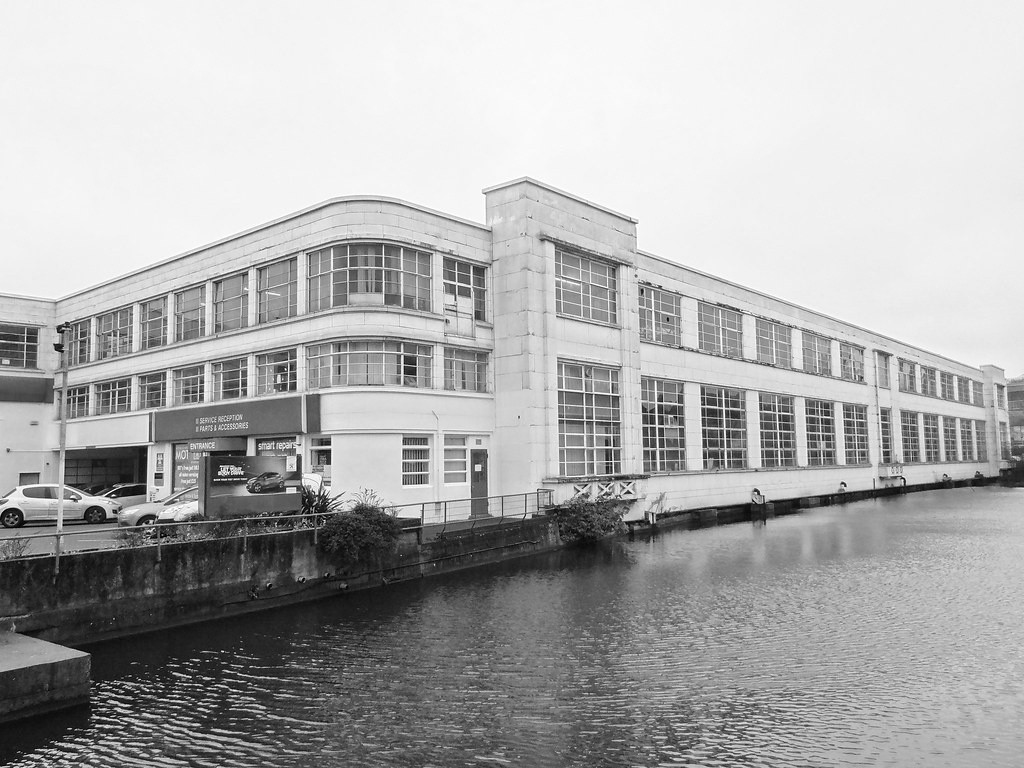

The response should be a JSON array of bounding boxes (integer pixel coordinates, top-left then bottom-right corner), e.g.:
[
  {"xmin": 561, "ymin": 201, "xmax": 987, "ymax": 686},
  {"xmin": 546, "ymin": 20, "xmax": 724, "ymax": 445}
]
[{"xmin": 52, "ymin": 322, "xmax": 71, "ymax": 545}]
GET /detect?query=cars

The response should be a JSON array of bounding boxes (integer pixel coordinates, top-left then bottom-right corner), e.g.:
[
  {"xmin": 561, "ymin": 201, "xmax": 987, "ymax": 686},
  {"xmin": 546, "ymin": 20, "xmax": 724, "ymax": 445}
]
[
  {"xmin": 246, "ymin": 471, "xmax": 284, "ymax": 494},
  {"xmin": 92, "ymin": 483, "xmax": 148, "ymax": 510},
  {"xmin": 119, "ymin": 484, "xmax": 199, "ymax": 536},
  {"xmin": 0, "ymin": 483, "xmax": 122, "ymax": 529}
]
